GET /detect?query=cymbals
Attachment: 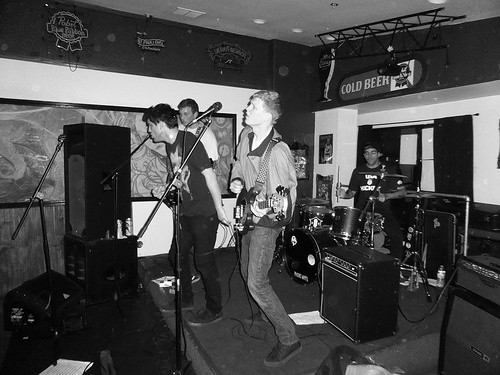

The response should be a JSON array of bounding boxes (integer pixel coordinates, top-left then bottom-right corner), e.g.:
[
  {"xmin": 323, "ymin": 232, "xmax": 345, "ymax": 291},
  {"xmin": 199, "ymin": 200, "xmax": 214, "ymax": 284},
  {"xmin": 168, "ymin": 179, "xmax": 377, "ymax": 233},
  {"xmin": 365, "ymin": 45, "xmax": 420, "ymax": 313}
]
[
  {"xmin": 358, "ymin": 171, "xmax": 407, "ymax": 179},
  {"xmin": 297, "ymin": 197, "xmax": 329, "ymax": 205}
]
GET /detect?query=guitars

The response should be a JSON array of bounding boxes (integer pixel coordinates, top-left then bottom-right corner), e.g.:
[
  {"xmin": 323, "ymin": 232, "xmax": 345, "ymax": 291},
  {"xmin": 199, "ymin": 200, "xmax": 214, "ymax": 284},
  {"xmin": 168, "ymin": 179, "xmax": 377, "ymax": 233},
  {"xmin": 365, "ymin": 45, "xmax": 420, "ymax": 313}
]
[{"xmin": 233, "ymin": 182, "xmax": 289, "ymax": 234}]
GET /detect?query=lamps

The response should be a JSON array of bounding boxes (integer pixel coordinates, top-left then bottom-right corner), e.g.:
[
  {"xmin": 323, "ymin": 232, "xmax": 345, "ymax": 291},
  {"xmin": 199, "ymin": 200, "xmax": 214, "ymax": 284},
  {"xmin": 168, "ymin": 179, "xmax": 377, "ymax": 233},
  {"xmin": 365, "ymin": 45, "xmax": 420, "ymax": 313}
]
[{"xmin": 376, "ymin": 53, "xmax": 401, "ymax": 76}]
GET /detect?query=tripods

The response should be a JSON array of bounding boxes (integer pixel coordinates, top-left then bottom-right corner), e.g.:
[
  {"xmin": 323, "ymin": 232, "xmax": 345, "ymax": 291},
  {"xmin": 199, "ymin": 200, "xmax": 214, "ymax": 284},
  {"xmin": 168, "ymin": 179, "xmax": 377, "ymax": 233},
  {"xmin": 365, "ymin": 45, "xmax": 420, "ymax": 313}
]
[
  {"xmin": 12, "ymin": 137, "xmax": 76, "ymax": 365},
  {"xmin": 399, "ymin": 200, "xmax": 433, "ymax": 304}
]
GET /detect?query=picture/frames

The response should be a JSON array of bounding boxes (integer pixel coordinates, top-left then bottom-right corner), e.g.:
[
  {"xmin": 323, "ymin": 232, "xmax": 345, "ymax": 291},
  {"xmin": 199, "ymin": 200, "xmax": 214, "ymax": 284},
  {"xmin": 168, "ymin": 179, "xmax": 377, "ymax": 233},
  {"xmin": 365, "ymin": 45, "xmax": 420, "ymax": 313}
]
[
  {"xmin": 0, "ymin": 97, "xmax": 237, "ymax": 209},
  {"xmin": 288, "ymin": 141, "xmax": 311, "ymax": 181}
]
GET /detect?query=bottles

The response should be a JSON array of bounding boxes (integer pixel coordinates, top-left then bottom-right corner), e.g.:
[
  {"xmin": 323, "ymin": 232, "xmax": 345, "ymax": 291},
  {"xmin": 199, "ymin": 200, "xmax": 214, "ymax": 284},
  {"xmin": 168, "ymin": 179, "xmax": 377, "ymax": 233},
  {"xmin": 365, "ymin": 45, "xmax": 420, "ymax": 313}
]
[
  {"xmin": 436, "ymin": 266, "xmax": 446, "ymax": 287},
  {"xmin": 126, "ymin": 217, "xmax": 132, "ymax": 236},
  {"xmin": 324, "ymin": 138, "xmax": 332, "ymax": 160}
]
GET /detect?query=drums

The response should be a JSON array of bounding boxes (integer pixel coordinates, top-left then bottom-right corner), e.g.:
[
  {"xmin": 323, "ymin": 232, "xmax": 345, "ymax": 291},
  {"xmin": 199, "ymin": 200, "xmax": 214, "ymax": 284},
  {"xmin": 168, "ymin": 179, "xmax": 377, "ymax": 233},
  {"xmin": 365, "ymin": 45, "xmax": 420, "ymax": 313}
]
[
  {"xmin": 329, "ymin": 206, "xmax": 365, "ymax": 241},
  {"xmin": 303, "ymin": 207, "xmax": 335, "ymax": 231},
  {"xmin": 283, "ymin": 228, "xmax": 339, "ymax": 285}
]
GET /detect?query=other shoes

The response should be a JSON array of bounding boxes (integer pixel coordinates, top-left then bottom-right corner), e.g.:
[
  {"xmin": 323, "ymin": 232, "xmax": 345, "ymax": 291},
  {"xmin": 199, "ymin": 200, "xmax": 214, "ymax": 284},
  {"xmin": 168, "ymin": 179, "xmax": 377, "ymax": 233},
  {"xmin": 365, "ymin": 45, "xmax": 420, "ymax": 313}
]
[
  {"xmin": 188, "ymin": 308, "xmax": 222, "ymax": 325},
  {"xmin": 264, "ymin": 341, "xmax": 303, "ymax": 367},
  {"xmin": 246, "ymin": 312, "xmax": 272, "ymax": 326},
  {"xmin": 160, "ymin": 298, "xmax": 193, "ymax": 311}
]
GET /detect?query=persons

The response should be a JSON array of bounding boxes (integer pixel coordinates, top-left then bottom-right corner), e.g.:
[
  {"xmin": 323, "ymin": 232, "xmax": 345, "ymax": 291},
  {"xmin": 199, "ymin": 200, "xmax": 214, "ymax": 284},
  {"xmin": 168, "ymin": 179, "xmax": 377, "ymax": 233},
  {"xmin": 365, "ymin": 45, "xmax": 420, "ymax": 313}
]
[
  {"xmin": 141, "ymin": 103, "xmax": 231, "ymax": 326},
  {"xmin": 228, "ymin": 90, "xmax": 303, "ymax": 367},
  {"xmin": 336, "ymin": 142, "xmax": 406, "ymax": 262},
  {"xmin": 166, "ymin": 98, "xmax": 219, "ymax": 294}
]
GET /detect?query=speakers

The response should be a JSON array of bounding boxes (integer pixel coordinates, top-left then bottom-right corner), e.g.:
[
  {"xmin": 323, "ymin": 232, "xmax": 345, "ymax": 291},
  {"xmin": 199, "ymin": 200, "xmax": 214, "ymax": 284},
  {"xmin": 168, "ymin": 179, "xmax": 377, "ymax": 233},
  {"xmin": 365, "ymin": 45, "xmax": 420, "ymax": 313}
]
[
  {"xmin": 64, "ymin": 233, "xmax": 138, "ymax": 306},
  {"xmin": 3, "ymin": 270, "xmax": 84, "ymax": 337},
  {"xmin": 437, "ymin": 283, "xmax": 500, "ymax": 375},
  {"xmin": 63, "ymin": 123, "xmax": 131, "ymax": 240}
]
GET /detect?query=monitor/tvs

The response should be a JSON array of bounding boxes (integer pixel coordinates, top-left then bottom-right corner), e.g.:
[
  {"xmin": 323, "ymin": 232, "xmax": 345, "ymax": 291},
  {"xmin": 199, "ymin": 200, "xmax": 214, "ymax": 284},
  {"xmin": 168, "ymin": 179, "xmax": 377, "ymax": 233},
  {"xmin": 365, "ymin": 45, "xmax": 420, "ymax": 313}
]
[{"xmin": 320, "ymin": 244, "xmax": 400, "ymax": 343}]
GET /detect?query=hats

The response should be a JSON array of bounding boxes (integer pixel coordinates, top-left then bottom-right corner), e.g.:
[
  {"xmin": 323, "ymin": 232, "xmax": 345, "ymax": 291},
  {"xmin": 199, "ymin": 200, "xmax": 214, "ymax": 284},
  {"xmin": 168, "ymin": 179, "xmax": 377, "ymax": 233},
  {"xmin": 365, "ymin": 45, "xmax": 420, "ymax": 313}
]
[{"xmin": 362, "ymin": 142, "xmax": 380, "ymax": 151}]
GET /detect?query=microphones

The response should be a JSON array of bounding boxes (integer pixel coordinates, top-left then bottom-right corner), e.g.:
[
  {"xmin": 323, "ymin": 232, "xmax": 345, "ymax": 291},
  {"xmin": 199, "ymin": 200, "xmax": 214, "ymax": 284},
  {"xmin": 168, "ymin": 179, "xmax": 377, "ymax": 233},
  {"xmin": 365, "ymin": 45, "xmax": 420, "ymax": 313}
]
[{"xmin": 188, "ymin": 102, "xmax": 222, "ymax": 128}]
